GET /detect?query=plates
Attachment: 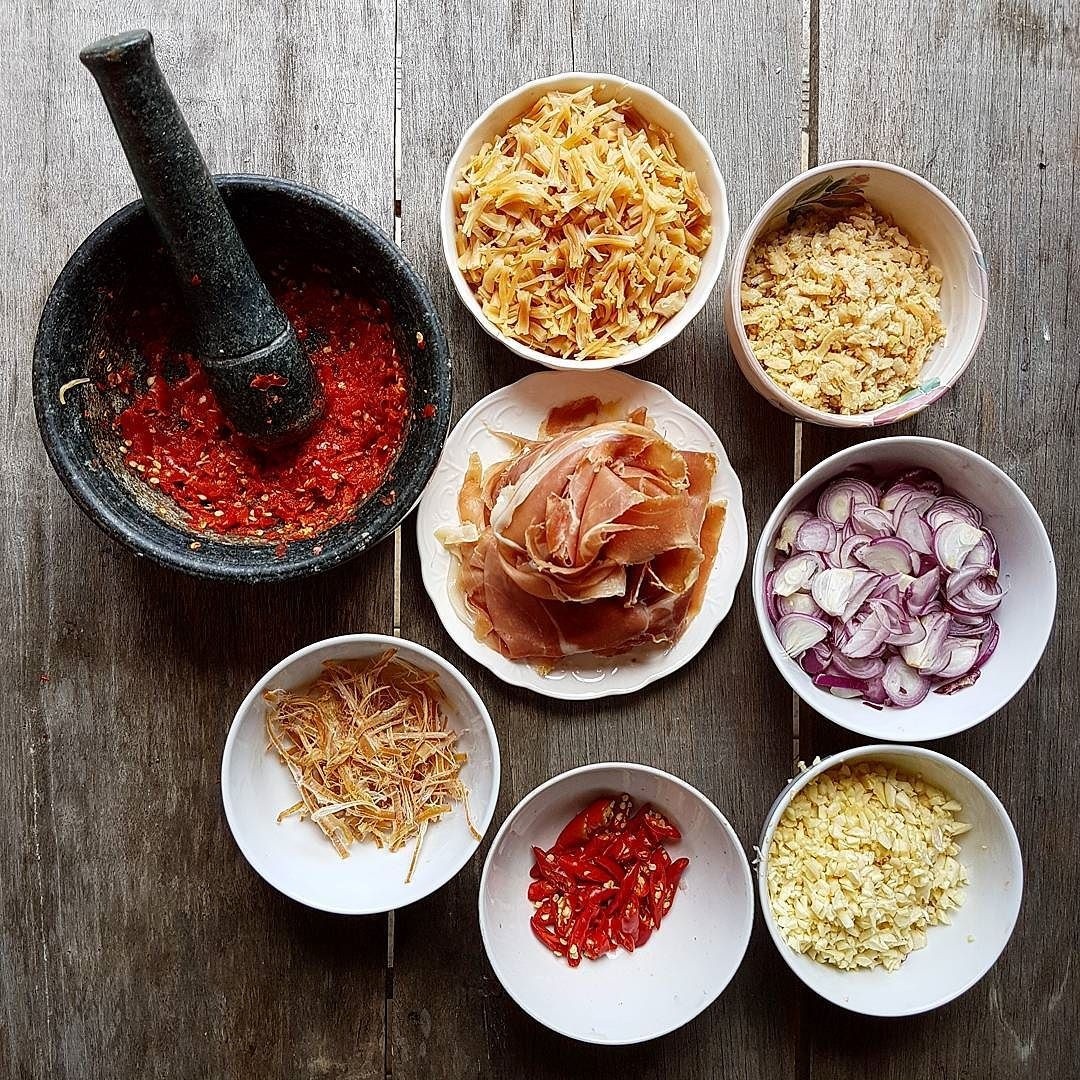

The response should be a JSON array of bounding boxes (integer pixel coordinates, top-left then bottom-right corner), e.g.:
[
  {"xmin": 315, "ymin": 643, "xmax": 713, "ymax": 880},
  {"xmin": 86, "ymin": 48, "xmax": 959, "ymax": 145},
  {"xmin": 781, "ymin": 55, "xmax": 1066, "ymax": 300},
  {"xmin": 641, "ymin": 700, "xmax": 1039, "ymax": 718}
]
[{"xmin": 415, "ymin": 370, "xmax": 749, "ymax": 702}]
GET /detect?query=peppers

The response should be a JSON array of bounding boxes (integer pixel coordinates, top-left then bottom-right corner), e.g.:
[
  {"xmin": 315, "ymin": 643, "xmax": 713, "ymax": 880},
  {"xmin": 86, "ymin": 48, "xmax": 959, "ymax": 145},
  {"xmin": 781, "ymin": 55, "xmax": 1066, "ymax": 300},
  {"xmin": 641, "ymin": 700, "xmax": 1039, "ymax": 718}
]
[{"xmin": 527, "ymin": 793, "xmax": 689, "ymax": 967}]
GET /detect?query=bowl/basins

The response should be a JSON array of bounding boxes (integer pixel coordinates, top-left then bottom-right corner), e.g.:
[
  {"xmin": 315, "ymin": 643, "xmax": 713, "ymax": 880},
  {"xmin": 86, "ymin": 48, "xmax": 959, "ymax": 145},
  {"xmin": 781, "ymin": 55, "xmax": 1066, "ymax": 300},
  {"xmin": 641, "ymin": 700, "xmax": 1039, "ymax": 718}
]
[
  {"xmin": 440, "ymin": 72, "xmax": 731, "ymax": 372},
  {"xmin": 751, "ymin": 434, "xmax": 1057, "ymax": 745},
  {"xmin": 725, "ymin": 157, "xmax": 993, "ymax": 425},
  {"xmin": 219, "ymin": 635, "xmax": 502, "ymax": 914},
  {"xmin": 29, "ymin": 174, "xmax": 458, "ymax": 586},
  {"xmin": 477, "ymin": 763, "xmax": 756, "ymax": 1043},
  {"xmin": 755, "ymin": 744, "xmax": 1025, "ymax": 1019}
]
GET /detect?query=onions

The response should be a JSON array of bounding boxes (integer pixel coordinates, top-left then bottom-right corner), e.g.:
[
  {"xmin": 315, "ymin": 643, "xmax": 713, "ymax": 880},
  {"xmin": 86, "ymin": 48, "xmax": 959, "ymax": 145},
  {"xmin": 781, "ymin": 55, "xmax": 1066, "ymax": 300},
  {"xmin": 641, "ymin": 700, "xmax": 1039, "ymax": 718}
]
[{"xmin": 766, "ymin": 464, "xmax": 1006, "ymax": 710}]
